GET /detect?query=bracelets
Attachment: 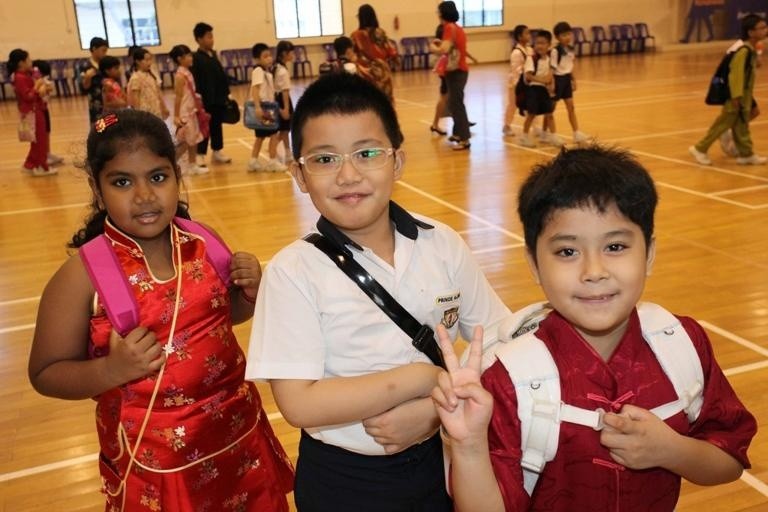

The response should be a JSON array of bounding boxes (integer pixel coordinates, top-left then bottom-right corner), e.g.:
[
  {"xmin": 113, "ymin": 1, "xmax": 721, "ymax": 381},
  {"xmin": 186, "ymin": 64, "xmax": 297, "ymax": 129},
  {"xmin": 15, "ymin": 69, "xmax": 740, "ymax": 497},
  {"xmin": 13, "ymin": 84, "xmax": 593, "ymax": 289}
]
[{"xmin": 240, "ymin": 293, "xmax": 256, "ymax": 305}]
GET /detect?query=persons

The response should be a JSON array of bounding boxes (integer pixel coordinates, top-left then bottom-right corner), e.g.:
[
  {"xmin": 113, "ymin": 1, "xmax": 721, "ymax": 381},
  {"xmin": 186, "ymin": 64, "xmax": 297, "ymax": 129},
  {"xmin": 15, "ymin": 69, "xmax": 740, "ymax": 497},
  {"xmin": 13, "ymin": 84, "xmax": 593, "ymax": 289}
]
[
  {"xmin": 7, "ymin": 49, "xmax": 58, "ymax": 176},
  {"xmin": 32, "ymin": 58, "xmax": 63, "ymax": 165},
  {"xmin": 539, "ymin": 21, "xmax": 586, "ymax": 143},
  {"xmin": 247, "ymin": 43, "xmax": 287, "ymax": 172},
  {"xmin": 426, "ymin": 1, "xmax": 468, "ymax": 153},
  {"xmin": 272, "ymin": 40, "xmax": 296, "ymax": 164},
  {"xmin": 78, "ymin": 37, "xmax": 129, "ymax": 171},
  {"xmin": 427, "ymin": 22, "xmax": 477, "ymax": 134},
  {"xmin": 689, "ymin": 15, "xmax": 767, "ymax": 166},
  {"xmin": 191, "ymin": 23, "xmax": 233, "ymax": 166},
  {"xmin": 124, "ymin": 46, "xmax": 169, "ymax": 121},
  {"xmin": 331, "ymin": 4, "xmax": 397, "ymax": 100},
  {"xmin": 244, "ymin": 71, "xmax": 513, "ymax": 512},
  {"xmin": 27, "ymin": 108, "xmax": 294, "ymax": 512},
  {"xmin": 170, "ymin": 44, "xmax": 209, "ymax": 173},
  {"xmin": 502, "ymin": 25, "xmax": 535, "ymax": 137},
  {"xmin": 431, "ymin": 142, "xmax": 758, "ymax": 512},
  {"xmin": 518, "ymin": 29, "xmax": 565, "ymax": 148}
]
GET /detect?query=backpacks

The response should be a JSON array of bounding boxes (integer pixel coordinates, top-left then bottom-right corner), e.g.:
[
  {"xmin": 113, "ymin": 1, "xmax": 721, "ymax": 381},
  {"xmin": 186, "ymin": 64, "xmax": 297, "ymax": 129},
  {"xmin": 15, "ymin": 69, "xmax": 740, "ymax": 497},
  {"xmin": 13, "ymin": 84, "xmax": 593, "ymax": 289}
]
[
  {"xmin": 319, "ymin": 58, "xmax": 349, "ymax": 76},
  {"xmin": 440, "ymin": 300, "xmax": 706, "ymax": 502}
]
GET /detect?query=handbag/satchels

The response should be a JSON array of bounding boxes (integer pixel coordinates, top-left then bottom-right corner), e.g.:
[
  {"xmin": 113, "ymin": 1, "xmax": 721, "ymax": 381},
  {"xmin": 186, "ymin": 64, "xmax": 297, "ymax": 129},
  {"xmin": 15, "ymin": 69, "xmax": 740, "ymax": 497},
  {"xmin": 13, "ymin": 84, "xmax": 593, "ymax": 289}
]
[
  {"xmin": 446, "ymin": 48, "xmax": 460, "ymax": 71},
  {"xmin": 705, "ymin": 54, "xmax": 730, "ymax": 104},
  {"xmin": 222, "ymin": 98, "xmax": 240, "ymax": 124},
  {"xmin": 515, "ymin": 74, "xmax": 527, "ymax": 110},
  {"xmin": 16, "ymin": 112, "xmax": 36, "ymax": 141},
  {"xmin": 197, "ymin": 110, "xmax": 212, "ymax": 139},
  {"xmin": 243, "ymin": 101, "xmax": 281, "ymax": 132}
]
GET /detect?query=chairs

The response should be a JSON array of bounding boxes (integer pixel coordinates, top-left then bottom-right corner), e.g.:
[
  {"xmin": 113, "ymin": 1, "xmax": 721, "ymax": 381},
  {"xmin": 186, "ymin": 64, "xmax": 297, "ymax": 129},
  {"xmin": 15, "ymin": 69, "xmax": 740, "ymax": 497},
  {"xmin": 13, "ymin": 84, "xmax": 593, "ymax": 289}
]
[
  {"xmin": 0, "ymin": 44, "xmax": 315, "ymax": 99},
  {"xmin": 510, "ymin": 22, "xmax": 655, "ymax": 57},
  {"xmin": 321, "ymin": 38, "xmax": 435, "ymax": 71}
]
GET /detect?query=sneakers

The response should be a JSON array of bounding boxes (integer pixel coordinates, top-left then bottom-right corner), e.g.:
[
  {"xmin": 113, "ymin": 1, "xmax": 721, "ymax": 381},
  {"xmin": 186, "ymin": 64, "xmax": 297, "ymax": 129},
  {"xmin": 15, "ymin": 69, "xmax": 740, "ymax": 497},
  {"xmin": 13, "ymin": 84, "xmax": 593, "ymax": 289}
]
[
  {"xmin": 573, "ymin": 131, "xmax": 591, "ymax": 143},
  {"xmin": 32, "ymin": 151, "xmax": 63, "ymax": 176},
  {"xmin": 248, "ymin": 158, "xmax": 293, "ymax": 172},
  {"xmin": 178, "ymin": 152, "xmax": 231, "ymax": 175},
  {"xmin": 688, "ymin": 128, "xmax": 766, "ymax": 165},
  {"xmin": 519, "ymin": 127, "xmax": 564, "ymax": 148},
  {"xmin": 504, "ymin": 126, "xmax": 513, "ymax": 135},
  {"xmin": 431, "ymin": 121, "xmax": 476, "ymax": 149}
]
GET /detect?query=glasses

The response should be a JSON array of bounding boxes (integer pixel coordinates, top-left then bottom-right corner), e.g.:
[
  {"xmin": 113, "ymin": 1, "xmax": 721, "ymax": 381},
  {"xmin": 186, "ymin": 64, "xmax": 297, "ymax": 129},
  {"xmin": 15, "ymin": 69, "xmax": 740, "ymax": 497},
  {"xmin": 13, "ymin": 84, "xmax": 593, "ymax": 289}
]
[{"xmin": 297, "ymin": 148, "xmax": 393, "ymax": 176}]
